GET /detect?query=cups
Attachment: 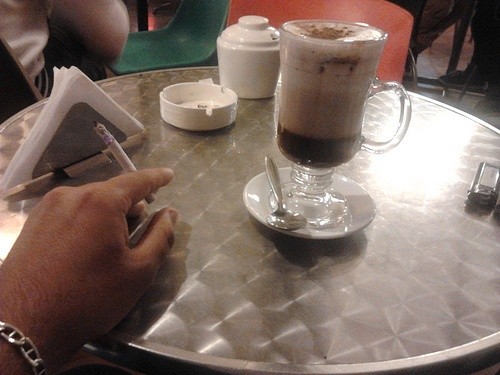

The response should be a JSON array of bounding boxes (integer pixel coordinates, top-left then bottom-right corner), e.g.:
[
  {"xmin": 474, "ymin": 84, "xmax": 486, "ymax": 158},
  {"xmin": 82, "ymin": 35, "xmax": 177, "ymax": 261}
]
[{"xmin": 216, "ymin": 14, "xmax": 282, "ymax": 100}]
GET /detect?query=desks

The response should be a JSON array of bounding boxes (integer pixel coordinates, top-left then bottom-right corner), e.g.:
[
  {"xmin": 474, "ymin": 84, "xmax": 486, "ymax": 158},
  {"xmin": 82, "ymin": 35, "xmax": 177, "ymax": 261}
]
[{"xmin": 0, "ymin": 65, "xmax": 500, "ymax": 375}]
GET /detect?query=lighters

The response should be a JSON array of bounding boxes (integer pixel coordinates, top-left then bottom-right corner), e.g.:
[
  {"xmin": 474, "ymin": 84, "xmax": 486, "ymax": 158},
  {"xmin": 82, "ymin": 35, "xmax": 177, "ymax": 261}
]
[{"xmin": 466, "ymin": 161, "xmax": 500, "ymax": 208}]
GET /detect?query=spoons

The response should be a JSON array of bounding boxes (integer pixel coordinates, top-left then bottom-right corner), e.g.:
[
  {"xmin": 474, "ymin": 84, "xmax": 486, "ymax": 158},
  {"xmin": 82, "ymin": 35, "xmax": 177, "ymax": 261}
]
[{"xmin": 264, "ymin": 155, "xmax": 307, "ymax": 230}]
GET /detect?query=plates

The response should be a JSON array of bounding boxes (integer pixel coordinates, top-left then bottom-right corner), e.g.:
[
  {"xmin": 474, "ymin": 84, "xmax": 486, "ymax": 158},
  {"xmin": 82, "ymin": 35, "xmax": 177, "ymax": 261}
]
[{"xmin": 243, "ymin": 166, "xmax": 377, "ymax": 237}]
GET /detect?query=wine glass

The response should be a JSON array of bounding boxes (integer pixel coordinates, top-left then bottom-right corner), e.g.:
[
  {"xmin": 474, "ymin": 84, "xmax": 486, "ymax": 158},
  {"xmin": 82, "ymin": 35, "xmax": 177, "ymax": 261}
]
[{"xmin": 269, "ymin": 19, "xmax": 412, "ymax": 229}]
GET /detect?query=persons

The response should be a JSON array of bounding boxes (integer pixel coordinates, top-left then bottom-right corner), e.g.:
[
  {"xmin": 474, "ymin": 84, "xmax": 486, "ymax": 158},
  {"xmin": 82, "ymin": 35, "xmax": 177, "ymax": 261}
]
[
  {"xmin": 0, "ymin": 0, "xmax": 131, "ymax": 129},
  {"xmin": 439, "ymin": 0, "xmax": 500, "ymax": 117},
  {"xmin": 0, "ymin": 168, "xmax": 178, "ymax": 375}
]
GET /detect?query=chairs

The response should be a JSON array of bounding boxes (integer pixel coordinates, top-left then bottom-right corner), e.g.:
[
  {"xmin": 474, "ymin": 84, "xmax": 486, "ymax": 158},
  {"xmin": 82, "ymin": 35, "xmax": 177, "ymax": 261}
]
[
  {"xmin": 107, "ymin": 0, "xmax": 229, "ymax": 76},
  {"xmin": 227, "ymin": 1, "xmax": 414, "ymax": 83}
]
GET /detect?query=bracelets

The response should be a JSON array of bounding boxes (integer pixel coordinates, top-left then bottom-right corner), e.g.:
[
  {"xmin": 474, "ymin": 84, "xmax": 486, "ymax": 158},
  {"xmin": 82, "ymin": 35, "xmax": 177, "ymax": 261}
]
[{"xmin": 0, "ymin": 320, "xmax": 46, "ymax": 375}]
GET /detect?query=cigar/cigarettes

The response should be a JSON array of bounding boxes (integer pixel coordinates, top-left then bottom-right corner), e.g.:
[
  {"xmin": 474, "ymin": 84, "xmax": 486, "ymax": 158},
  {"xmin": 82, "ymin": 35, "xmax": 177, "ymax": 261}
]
[{"xmin": 93, "ymin": 120, "xmax": 156, "ymax": 204}]
